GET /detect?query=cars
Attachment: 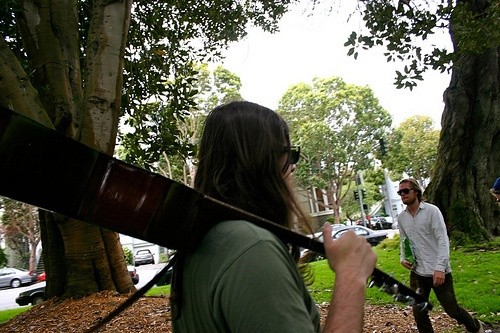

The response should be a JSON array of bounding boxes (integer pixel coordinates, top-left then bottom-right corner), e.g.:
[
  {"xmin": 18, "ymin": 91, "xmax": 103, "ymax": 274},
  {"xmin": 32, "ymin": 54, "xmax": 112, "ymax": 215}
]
[
  {"xmin": 299, "ymin": 216, "xmax": 399, "ymax": 264},
  {"xmin": 0, "ymin": 268, "xmax": 46, "ymax": 290},
  {"xmin": 15, "ymin": 266, "xmax": 140, "ymax": 306},
  {"xmin": 133, "ymin": 248, "xmax": 155, "ymax": 267}
]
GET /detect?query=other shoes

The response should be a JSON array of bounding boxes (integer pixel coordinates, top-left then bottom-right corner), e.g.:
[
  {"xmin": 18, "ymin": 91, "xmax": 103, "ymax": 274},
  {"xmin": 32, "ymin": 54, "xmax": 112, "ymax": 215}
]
[{"xmin": 476, "ymin": 320, "xmax": 484, "ymax": 333}]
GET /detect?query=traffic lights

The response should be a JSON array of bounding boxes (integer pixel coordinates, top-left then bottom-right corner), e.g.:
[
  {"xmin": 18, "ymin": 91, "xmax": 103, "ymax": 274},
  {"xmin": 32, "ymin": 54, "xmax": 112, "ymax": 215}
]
[
  {"xmin": 353, "ymin": 190, "xmax": 360, "ymax": 200},
  {"xmin": 362, "ymin": 190, "xmax": 367, "ymax": 199}
]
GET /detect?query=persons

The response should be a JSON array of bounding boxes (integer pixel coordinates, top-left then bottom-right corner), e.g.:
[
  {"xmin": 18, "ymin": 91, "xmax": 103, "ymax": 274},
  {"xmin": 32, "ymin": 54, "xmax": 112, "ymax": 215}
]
[
  {"xmin": 397, "ymin": 178, "xmax": 483, "ymax": 333},
  {"xmin": 490, "ymin": 177, "xmax": 500, "ymax": 202},
  {"xmin": 170, "ymin": 100, "xmax": 376, "ymax": 333}
]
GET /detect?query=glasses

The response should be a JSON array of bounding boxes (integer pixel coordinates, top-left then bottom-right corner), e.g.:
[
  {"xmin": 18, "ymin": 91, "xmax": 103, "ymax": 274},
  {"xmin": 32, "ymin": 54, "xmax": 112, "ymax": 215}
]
[
  {"xmin": 397, "ymin": 188, "xmax": 414, "ymax": 195},
  {"xmin": 290, "ymin": 146, "xmax": 300, "ymax": 164}
]
